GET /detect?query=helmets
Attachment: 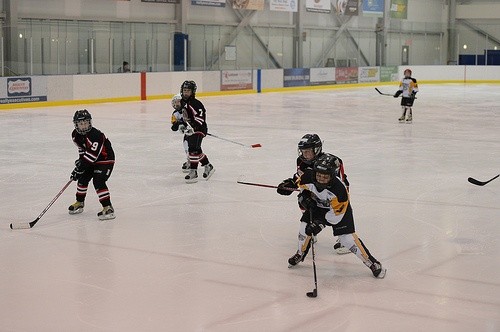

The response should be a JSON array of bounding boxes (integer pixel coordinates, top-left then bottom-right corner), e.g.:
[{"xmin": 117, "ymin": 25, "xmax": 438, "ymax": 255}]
[
  {"xmin": 73, "ymin": 109, "xmax": 92, "ymax": 134},
  {"xmin": 181, "ymin": 80, "xmax": 197, "ymax": 100},
  {"xmin": 314, "ymin": 154, "xmax": 340, "ymax": 184},
  {"xmin": 172, "ymin": 94, "xmax": 182, "ymax": 109},
  {"xmin": 297, "ymin": 133, "xmax": 322, "ymax": 163}
]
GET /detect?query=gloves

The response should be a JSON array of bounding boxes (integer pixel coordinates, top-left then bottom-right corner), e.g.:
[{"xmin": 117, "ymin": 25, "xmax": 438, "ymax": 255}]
[
  {"xmin": 277, "ymin": 178, "xmax": 297, "ymax": 195},
  {"xmin": 394, "ymin": 92, "xmax": 399, "ymax": 97},
  {"xmin": 410, "ymin": 92, "xmax": 415, "ymax": 99},
  {"xmin": 70, "ymin": 159, "xmax": 85, "ymax": 180},
  {"xmin": 305, "ymin": 217, "xmax": 326, "ymax": 236},
  {"xmin": 298, "ymin": 189, "xmax": 316, "ymax": 209}
]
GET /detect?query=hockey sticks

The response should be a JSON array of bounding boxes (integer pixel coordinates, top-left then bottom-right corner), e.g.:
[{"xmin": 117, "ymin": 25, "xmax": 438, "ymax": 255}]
[
  {"xmin": 375, "ymin": 87, "xmax": 417, "ymax": 99},
  {"xmin": 207, "ymin": 133, "xmax": 262, "ymax": 148},
  {"xmin": 10, "ymin": 179, "xmax": 73, "ymax": 229},
  {"xmin": 306, "ymin": 207, "xmax": 318, "ymax": 298},
  {"xmin": 236, "ymin": 174, "xmax": 301, "ymax": 192},
  {"xmin": 468, "ymin": 174, "xmax": 500, "ymax": 186}
]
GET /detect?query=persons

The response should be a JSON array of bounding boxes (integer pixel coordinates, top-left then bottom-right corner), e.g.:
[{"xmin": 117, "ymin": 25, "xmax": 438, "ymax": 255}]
[
  {"xmin": 119, "ymin": 62, "xmax": 130, "ymax": 72},
  {"xmin": 170, "ymin": 80, "xmax": 215, "ymax": 184},
  {"xmin": 68, "ymin": 109, "xmax": 115, "ymax": 220},
  {"xmin": 395, "ymin": 69, "xmax": 419, "ymax": 124},
  {"xmin": 278, "ymin": 134, "xmax": 352, "ymax": 255},
  {"xmin": 288, "ymin": 153, "xmax": 386, "ymax": 278}
]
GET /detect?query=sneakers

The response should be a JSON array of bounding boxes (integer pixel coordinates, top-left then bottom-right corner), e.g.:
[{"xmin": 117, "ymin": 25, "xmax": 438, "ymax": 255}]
[
  {"xmin": 203, "ymin": 163, "xmax": 215, "ymax": 180},
  {"xmin": 370, "ymin": 260, "xmax": 386, "ymax": 279},
  {"xmin": 68, "ymin": 201, "xmax": 84, "ymax": 214},
  {"xmin": 185, "ymin": 170, "xmax": 198, "ymax": 183},
  {"xmin": 406, "ymin": 117, "xmax": 412, "ymax": 123},
  {"xmin": 399, "ymin": 116, "xmax": 405, "ymax": 122},
  {"xmin": 182, "ymin": 161, "xmax": 191, "ymax": 172},
  {"xmin": 334, "ymin": 242, "xmax": 351, "ymax": 254},
  {"xmin": 287, "ymin": 254, "xmax": 301, "ymax": 268},
  {"xmin": 98, "ymin": 205, "xmax": 115, "ymax": 220}
]
